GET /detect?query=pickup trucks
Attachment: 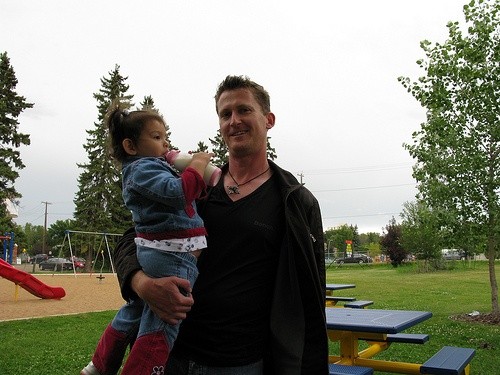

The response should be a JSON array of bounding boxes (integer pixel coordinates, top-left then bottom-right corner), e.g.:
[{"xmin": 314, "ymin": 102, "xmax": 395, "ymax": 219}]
[{"xmin": 30, "ymin": 253, "xmax": 54, "ymax": 263}]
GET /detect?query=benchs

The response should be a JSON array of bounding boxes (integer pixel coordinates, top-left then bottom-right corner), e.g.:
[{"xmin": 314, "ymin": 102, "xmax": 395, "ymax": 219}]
[{"xmin": 321, "ymin": 295, "xmax": 476, "ymax": 375}]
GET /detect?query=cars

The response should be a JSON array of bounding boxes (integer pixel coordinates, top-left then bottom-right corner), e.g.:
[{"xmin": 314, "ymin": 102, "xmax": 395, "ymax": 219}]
[
  {"xmin": 38, "ymin": 257, "xmax": 73, "ymax": 271},
  {"xmin": 335, "ymin": 254, "xmax": 373, "ymax": 264},
  {"xmin": 67, "ymin": 256, "xmax": 90, "ymax": 271},
  {"xmin": 441, "ymin": 248, "xmax": 474, "ymax": 261}
]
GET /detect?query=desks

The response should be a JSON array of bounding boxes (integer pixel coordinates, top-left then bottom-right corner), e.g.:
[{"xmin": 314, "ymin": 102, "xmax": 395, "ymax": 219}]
[
  {"xmin": 326, "ymin": 284, "xmax": 357, "ymax": 297},
  {"xmin": 325, "ymin": 307, "xmax": 433, "ymax": 358}
]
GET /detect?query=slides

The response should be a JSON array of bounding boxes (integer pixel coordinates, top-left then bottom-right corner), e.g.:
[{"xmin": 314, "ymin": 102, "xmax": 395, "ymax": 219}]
[{"xmin": 0, "ymin": 258, "xmax": 66, "ymax": 302}]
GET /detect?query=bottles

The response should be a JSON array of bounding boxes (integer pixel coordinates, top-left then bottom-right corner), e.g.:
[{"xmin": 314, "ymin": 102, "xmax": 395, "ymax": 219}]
[{"xmin": 164, "ymin": 149, "xmax": 222, "ymax": 186}]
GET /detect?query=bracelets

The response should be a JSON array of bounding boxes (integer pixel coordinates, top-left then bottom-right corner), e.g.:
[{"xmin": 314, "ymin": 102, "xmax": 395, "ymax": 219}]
[{"xmin": 226, "ymin": 165, "xmax": 270, "ymax": 194}]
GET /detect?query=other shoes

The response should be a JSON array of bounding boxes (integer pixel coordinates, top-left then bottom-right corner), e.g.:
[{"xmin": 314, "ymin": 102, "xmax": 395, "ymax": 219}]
[{"xmin": 80, "ymin": 360, "xmax": 101, "ymax": 375}]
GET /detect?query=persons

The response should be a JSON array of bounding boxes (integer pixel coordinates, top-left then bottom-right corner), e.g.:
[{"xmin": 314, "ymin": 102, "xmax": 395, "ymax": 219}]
[
  {"xmin": 80, "ymin": 108, "xmax": 216, "ymax": 375},
  {"xmin": 112, "ymin": 74, "xmax": 330, "ymax": 375}
]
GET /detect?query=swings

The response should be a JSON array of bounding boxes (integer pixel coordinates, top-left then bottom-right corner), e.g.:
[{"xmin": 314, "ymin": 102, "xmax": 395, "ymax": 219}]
[{"xmin": 79, "ymin": 233, "xmax": 95, "ymax": 273}]
